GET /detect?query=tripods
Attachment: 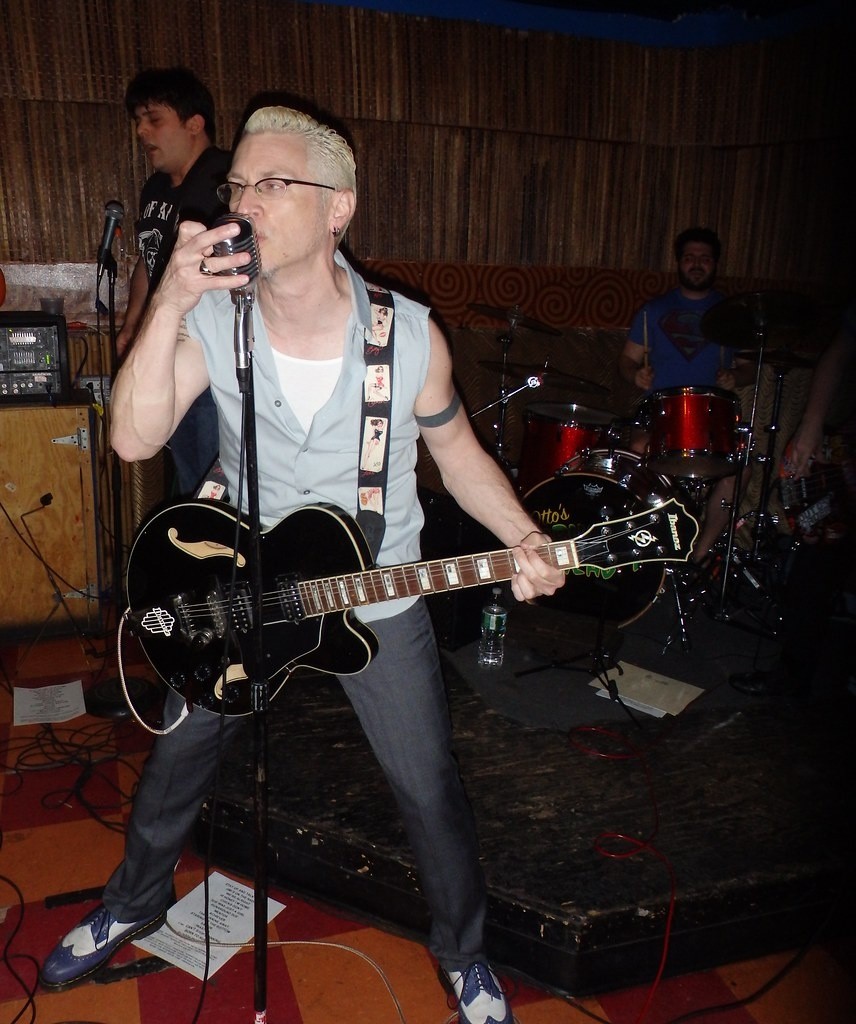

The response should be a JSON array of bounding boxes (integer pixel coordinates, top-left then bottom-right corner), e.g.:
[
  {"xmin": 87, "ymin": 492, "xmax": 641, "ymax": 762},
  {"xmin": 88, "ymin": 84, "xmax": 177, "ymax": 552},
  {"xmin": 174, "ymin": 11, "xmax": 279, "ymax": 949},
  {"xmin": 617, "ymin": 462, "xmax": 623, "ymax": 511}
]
[
  {"xmin": 514, "ymin": 577, "xmax": 647, "ymax": 733},
  {"xmin": 683, "ymin": 319, "xmax": 801, "ymax": 640}
]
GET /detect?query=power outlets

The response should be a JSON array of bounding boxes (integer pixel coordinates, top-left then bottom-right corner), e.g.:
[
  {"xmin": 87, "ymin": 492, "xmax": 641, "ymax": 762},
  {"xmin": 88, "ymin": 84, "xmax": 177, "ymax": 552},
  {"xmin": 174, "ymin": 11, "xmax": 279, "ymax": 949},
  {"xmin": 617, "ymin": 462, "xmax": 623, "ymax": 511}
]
[{"xmin": 80, "ymin": 376, "xmax": 110, "ymax": 406}]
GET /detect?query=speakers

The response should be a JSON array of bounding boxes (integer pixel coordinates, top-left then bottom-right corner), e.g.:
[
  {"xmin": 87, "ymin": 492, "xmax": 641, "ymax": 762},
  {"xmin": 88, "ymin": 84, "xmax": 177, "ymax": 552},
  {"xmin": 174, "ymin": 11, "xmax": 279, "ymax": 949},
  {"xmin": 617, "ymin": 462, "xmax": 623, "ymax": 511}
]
[{"xmin": 416, "ymin": 485, "xmax": 502, "ymax": 649}]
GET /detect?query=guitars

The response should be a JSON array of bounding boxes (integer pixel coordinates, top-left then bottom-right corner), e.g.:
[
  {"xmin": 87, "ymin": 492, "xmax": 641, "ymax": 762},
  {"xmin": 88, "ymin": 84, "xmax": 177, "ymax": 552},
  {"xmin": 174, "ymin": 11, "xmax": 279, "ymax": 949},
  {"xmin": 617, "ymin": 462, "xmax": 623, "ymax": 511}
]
[
  {"xmin": 125, "ymin": 497, "xmax": 701, "ymax": 718},
  {"xmin": 780, "ymin": 431, "xmax": 856, "ymax": 548}
]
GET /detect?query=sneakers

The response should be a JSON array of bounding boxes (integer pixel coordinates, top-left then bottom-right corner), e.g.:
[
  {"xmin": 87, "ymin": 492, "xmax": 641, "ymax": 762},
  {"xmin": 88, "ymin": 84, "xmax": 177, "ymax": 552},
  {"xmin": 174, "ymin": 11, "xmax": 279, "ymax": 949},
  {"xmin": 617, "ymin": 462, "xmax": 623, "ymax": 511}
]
[
  {"xmin": 39, "ymin": 907, "xmax": 167, "ymax": 991},
  {"xmin": 440, "ymin": 961, "xmax": 515, "ymax": 1024}
]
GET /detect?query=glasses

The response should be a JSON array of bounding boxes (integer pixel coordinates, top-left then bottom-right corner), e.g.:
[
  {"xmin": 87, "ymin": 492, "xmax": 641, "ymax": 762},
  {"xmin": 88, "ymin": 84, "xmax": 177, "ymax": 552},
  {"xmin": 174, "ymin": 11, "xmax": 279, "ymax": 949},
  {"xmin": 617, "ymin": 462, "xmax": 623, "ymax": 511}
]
[{"xmin": 217, "ymin": 178, "xmax": 342, "ymax": 205}]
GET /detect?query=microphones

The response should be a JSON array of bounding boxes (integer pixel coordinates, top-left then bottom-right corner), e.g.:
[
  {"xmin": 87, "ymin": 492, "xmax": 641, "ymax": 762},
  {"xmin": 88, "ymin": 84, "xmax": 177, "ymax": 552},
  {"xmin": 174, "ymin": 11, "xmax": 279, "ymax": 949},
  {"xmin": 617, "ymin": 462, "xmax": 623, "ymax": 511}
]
[
  {"xmin": 96, "ymin": 200, "xmax": 125, "ymax": 280},
  {"xmin": 212, "ymin": 212, "xmax": 262, "ymax": 393}
]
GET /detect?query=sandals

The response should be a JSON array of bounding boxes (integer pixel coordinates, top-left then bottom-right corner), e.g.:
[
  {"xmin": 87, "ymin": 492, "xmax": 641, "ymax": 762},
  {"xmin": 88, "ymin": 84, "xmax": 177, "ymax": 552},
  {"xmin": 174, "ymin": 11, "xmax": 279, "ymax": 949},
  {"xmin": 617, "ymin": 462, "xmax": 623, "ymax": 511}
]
[{"xmin": 691, "ymin": 551, "xmax": 722, "ymax": 578}]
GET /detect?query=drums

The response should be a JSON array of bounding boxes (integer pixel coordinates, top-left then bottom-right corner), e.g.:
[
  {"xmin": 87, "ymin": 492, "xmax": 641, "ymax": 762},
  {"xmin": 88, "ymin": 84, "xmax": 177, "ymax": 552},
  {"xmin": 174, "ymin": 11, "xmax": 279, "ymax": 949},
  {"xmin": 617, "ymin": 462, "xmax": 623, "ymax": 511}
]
[
  {"xmin": 520, "ymin": 446, "xmax": 696, "ymax": 629},
  {"xmin": 515, "ymin": 402, "xmax": 624, "ymax": 494},
  {"xmin": 634, "ymin": 386, "xmax": 744, "ymax": 481}
]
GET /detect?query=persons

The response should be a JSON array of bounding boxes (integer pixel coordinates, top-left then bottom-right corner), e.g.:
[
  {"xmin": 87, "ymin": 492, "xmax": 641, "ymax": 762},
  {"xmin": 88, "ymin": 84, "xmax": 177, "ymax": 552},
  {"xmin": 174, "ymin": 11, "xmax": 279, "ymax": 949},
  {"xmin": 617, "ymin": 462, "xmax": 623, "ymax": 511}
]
[
  {"xmin": 618, "ymin": 227, "xmax": 757, "ymax": 589},
  {"xmin": 785, "ymin": 306, "xmax": 856, "ymax": 481},
  {"xmin": 41, "ymin": 107, "xmax": 567, "ymax": 1024},
  {"xmin": 115, "ymin": 69, "xmax": 229, "ymax": 499}
]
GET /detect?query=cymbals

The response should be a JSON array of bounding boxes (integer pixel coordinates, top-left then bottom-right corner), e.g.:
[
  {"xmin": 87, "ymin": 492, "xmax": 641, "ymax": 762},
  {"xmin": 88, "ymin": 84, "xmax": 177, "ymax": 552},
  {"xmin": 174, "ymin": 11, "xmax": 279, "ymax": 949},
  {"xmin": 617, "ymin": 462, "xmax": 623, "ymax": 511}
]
[
  {"xmin": 733, "ymin": 348, "xmax": 820, "ymax": 371},
  {"xmin": 465, "ymin": 301, "xmax": 564, "ymax": 339},
  {"xmin": 697, "ymin": 288, "xmax": 823, "ymax": 351},
  {"xmin": 475, "ymin": 359, "xmax": 613, "ymax": 400}
]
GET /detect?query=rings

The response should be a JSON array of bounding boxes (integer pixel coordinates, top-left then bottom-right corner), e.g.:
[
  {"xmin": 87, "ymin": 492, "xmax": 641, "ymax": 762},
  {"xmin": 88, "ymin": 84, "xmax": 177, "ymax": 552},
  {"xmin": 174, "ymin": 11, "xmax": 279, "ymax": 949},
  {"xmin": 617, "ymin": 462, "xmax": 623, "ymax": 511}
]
[{"xmin": 200, "ymin": 258, "xmax": 212, "ymax": 276}]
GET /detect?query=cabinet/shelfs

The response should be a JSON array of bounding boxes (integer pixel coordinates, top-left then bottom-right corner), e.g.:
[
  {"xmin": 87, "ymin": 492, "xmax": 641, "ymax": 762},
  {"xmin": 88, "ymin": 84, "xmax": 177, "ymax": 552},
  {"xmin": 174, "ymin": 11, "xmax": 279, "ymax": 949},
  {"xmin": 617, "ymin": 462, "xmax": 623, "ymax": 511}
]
[{"xmin": 0, "ymin": 396, "xmax": 104, "ymax": 642}]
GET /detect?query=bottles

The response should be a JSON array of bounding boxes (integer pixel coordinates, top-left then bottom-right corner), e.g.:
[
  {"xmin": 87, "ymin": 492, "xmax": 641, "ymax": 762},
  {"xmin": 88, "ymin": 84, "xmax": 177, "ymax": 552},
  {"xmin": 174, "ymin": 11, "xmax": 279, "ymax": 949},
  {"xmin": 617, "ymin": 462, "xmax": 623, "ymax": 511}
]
[{"xmin": 478, "ymin": 589, "xmax": 507, "ymax": 669}]
